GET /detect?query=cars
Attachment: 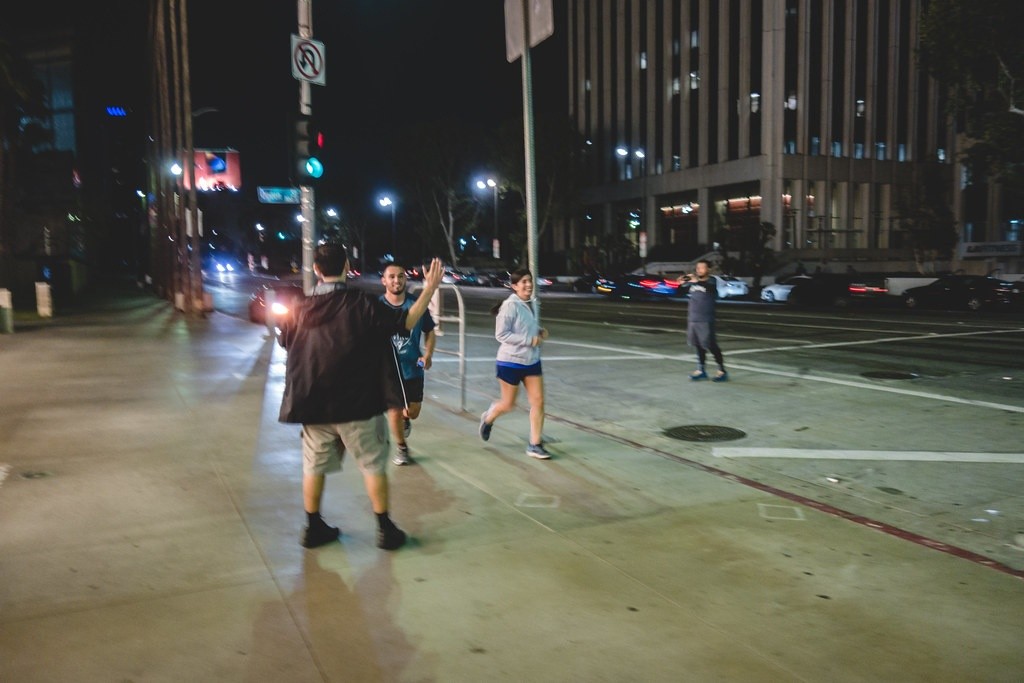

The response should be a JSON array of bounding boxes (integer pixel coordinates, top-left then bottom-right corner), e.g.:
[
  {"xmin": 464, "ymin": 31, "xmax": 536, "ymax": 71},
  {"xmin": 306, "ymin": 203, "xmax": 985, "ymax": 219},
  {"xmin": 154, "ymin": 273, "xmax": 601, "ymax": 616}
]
[
  {"xmin": 536, "ymin": 267, "xmax": 1024, "ymax": 322},
  {"xmin": 379, "ymin": 267, "xmax": 516, "ymax": 289},
  {"xmin": 247, "ymin": 285, "xmax": 304, "ymax": 324}
]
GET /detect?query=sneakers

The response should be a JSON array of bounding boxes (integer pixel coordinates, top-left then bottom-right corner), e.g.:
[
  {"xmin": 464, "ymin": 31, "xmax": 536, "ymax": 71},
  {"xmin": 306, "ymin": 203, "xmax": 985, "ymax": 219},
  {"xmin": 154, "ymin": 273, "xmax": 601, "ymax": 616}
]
[
  {"xmin": 402, "ymin": 416, "xmax": 412, "ymax": 437},
  {"xmin": 392, "ymin": 443, "xmax": 410, "ymax": 465},
  {"xmin": 526, "ymin": 442, "xmax": 552, "ymax": 459},
  {"xmin": 479, "ymin": 411, "xmax": 492, "ymax": 441}
]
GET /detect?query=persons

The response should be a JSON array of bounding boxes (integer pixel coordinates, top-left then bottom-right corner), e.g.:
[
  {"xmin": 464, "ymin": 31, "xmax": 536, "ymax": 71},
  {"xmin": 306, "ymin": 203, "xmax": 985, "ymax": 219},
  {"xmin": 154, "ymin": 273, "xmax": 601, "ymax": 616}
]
[
  {"xmin": 676, "ymin": 259, "xmax": 728, "ymax": 382},
  {"xmin": 480, "ymin": 267, "xmax": 553, "ymax": 458},
  {"xmin": 276, "ymin": 242, "xmax": 446, "ymax": 551},
  {"xmin": 377, "ymin": 264, "xmax": 436, "ymax": 466}
]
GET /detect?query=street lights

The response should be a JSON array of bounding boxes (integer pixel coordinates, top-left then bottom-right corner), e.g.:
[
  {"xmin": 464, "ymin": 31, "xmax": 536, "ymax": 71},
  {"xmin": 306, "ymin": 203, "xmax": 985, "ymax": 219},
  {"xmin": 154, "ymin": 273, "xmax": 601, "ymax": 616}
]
[
  {"xmin": 477, "ymin": 177, "xmax": 501, "ymax": 261},
  {"xmin": 378, "ymin": 195, "xmax": 397, "ymax": 265}
]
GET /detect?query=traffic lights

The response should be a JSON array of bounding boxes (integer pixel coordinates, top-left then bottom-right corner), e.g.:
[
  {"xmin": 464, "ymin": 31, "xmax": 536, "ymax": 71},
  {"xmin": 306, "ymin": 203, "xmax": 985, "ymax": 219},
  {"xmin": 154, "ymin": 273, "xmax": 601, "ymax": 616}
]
[{"xmin": 287, "ymin": 119, "xmax": 325, "ymax": 184}]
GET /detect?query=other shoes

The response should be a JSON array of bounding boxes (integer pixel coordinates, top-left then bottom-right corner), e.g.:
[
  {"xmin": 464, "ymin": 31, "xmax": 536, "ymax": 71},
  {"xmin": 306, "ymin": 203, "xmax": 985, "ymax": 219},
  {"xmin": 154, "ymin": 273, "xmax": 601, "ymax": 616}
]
[
  {"xmin": 689, "ymin": 370, "xmax": 707, "ymax": 380},
  {"xmin": 302, "ymin": 521, "xmax": 340, "ymax": 547},
  {"xmin": 376, "ymin": 524, "xmax": 406, "ymax": 550},
  {"xmin": 712, "ymin": 370, "xmax": 727, "ymax": 382}
]
